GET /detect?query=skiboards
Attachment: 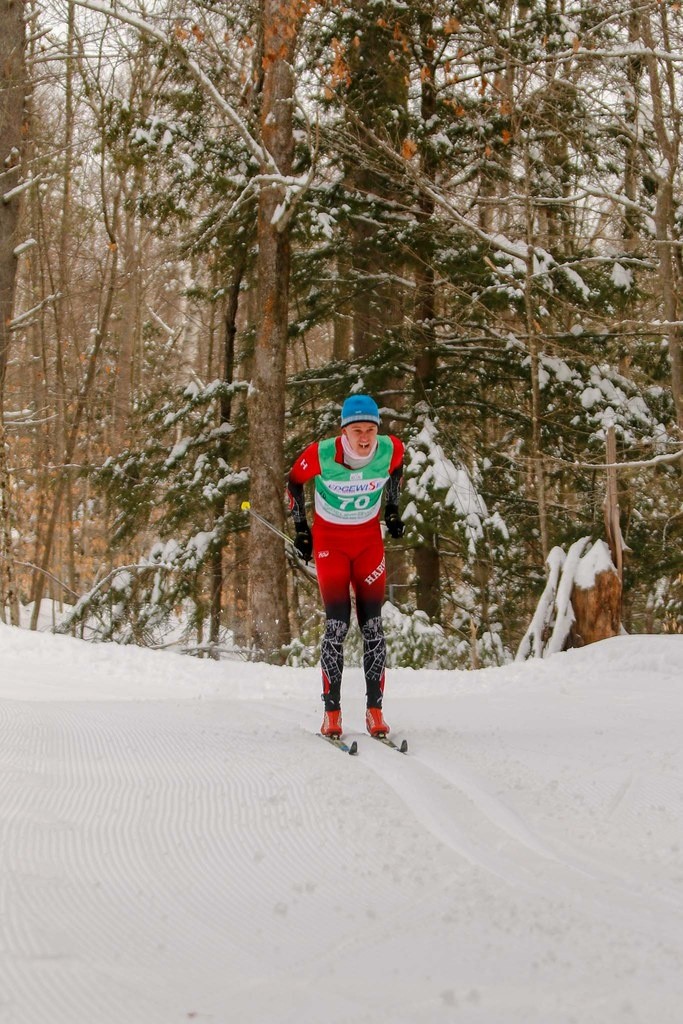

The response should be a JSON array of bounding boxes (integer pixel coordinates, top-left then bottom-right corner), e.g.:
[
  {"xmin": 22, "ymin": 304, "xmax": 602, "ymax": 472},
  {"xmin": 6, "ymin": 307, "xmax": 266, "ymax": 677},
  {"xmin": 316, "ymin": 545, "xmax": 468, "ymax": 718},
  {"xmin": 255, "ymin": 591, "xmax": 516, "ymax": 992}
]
[{"xmin": 314, "ymin": 730, "xmax": 408, "ymax": 755}]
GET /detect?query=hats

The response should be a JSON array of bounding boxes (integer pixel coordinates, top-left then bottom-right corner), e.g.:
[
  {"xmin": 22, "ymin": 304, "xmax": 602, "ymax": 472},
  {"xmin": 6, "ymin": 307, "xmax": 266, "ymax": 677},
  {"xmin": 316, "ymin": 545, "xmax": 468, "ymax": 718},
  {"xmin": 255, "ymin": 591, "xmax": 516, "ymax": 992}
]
[{"xmin": 341, "ymin": 395, "xmax": 380, "ymax": 428}]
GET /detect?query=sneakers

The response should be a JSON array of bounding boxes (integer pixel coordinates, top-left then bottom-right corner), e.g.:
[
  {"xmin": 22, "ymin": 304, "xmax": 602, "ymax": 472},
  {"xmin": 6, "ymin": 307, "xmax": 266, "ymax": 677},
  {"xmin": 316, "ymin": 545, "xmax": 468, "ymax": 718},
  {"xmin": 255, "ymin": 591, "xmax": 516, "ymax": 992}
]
[
  {"xmin": 366, "ymin": 707, "xmax": 389, "ymax": 735},
  {"xmin": 321, "ymin": 711, "xmax": 342, "ymax": 736}
]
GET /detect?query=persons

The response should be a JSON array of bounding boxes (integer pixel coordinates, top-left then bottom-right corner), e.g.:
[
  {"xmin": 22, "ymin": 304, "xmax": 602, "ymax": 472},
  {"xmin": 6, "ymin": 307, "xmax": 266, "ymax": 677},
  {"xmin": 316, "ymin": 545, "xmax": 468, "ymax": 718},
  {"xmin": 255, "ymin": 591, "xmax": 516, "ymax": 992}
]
[{"xmin": 286, "ymin": 395, "xmax": 406, "ymax": 738}]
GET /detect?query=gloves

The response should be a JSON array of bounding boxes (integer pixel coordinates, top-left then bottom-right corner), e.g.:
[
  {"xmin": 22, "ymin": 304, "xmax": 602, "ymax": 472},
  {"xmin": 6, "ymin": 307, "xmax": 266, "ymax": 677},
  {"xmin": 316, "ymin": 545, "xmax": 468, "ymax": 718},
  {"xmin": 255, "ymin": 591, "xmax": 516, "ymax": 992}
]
[
  {"xmin": 294, "ymin": 522, "xmax": 312, "ymax": 562},
  {"xmin": 385, "ymin": 504, "xmax": 406, "ymax": 539}
]
